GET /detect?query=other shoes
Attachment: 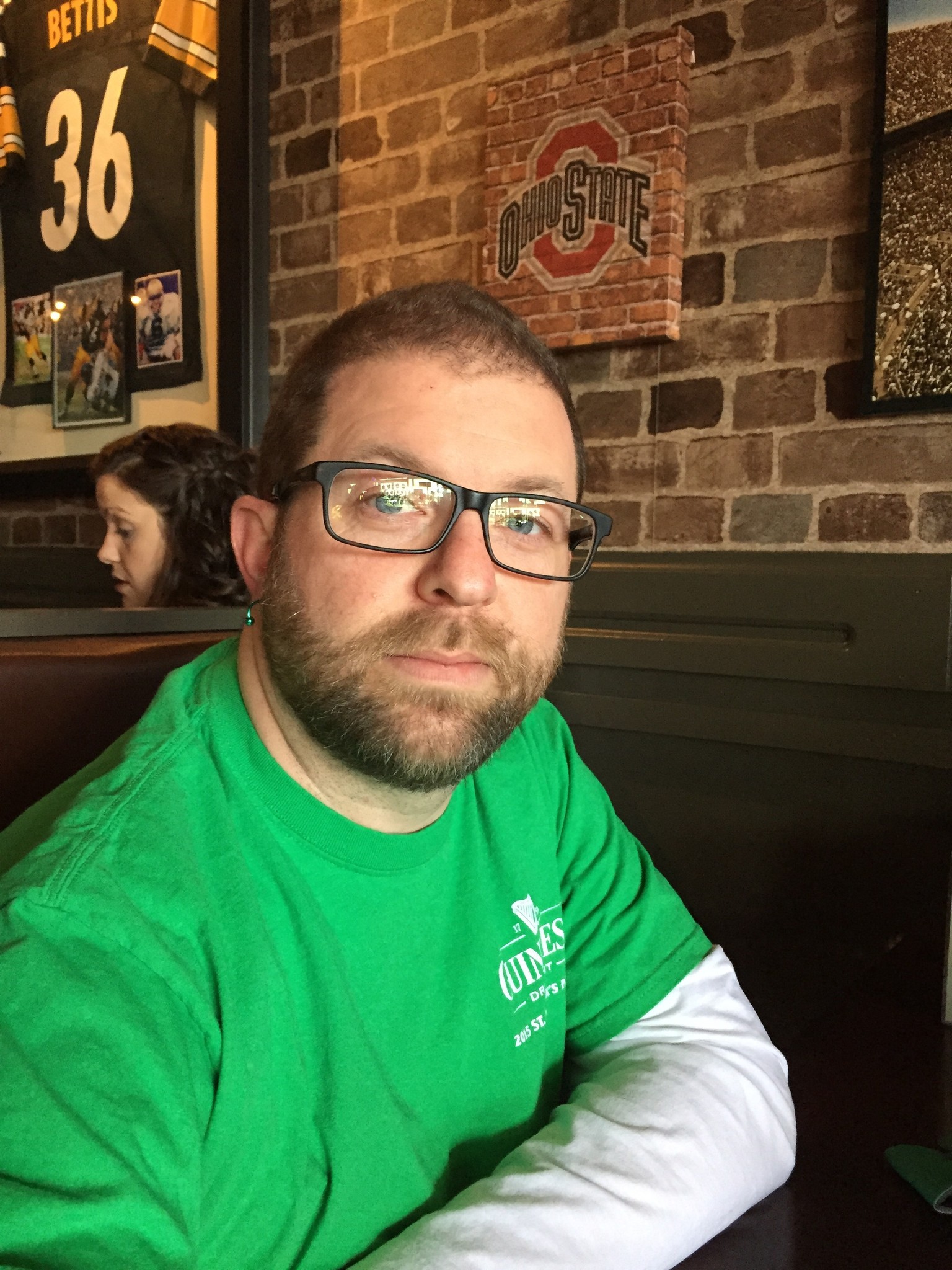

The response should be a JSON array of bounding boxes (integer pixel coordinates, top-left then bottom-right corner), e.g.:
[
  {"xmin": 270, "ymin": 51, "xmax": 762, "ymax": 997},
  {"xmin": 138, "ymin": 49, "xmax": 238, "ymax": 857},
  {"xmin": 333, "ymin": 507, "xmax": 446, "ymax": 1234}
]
[{"xmin": 58, "ymin": 408, "xmax": 66, "ymax": 417}]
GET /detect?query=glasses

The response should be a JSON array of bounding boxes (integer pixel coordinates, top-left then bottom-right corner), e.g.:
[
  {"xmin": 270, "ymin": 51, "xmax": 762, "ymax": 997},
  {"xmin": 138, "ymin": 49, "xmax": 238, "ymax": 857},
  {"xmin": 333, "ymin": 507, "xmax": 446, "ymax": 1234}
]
[
  {"xmin": 268, "ymin": 461, "xmax": 612, "ymax": 581},
  {"xmin": 148, "ymin": 294, "xmax": 163, "ymax": 301}
]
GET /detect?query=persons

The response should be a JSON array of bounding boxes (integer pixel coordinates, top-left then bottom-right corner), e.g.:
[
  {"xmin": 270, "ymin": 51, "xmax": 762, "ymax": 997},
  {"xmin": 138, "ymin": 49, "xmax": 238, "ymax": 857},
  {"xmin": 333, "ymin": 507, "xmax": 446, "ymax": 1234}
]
[
  {"xmin": 0, "ymin": 279, "xmax": 799, "ymax": 1270},
  {"xmin": 12, "ymin": 279, "xmax": 183, "ymax": 416},
  {"xmin": 88, "ymin": 421, "xmax": 263, "ymax": 613}
]
